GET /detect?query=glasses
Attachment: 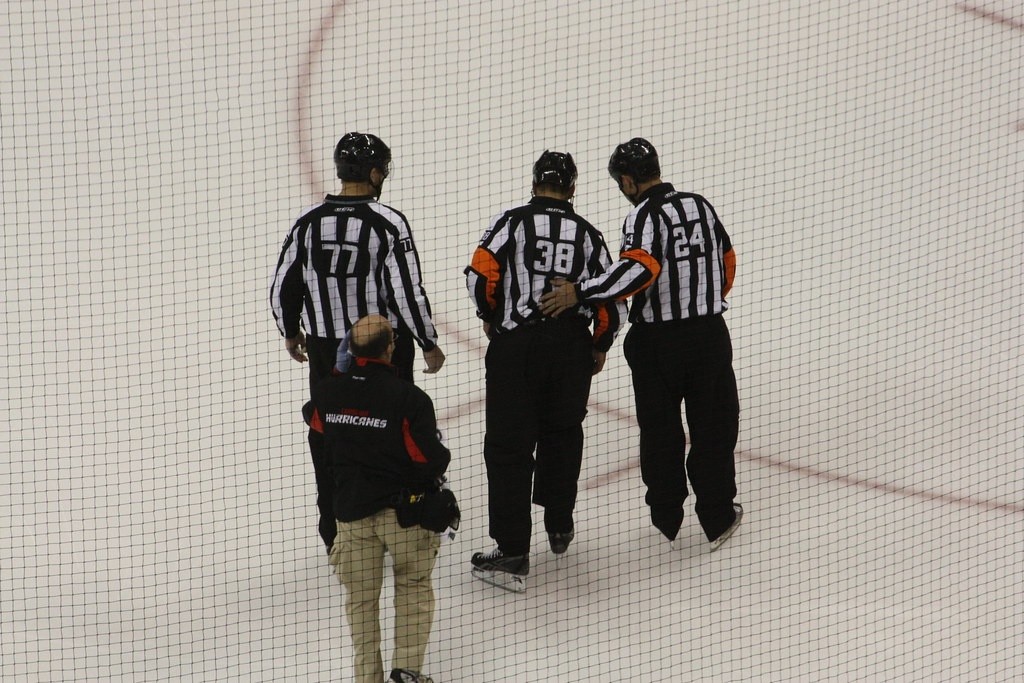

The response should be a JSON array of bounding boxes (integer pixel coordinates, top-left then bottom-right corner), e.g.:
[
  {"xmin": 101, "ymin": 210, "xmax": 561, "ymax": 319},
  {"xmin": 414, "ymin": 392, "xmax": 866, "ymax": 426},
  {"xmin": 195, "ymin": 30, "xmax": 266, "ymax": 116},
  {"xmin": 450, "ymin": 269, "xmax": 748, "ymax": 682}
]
[{"xmin": 390, "ymin": 333, "xmax": 400, "ymax": 341}]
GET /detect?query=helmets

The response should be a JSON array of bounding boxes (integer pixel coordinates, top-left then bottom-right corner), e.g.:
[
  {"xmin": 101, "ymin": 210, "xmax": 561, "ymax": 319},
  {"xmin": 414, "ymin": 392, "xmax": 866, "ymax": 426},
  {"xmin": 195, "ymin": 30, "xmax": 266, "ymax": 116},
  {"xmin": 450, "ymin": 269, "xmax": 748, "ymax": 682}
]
[
  {"xmin": 333, "ymin": 131, "xmax": 392, "ymax": 183},
  {"xmin": 531, "ymin": 149, "xmax": 579, "ymax": 191},
  {"xmin": 606, "ymin": 137, "xmax": 661, "ymax": 182}
]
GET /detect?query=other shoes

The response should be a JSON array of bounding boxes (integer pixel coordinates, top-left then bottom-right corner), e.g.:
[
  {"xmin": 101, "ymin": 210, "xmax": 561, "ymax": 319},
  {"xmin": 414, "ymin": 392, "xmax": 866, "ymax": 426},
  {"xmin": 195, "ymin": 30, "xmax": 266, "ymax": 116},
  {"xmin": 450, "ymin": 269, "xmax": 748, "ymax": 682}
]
[{"xmin": 387, "ymin": 668, "xmax": 432, "ymax": 683}]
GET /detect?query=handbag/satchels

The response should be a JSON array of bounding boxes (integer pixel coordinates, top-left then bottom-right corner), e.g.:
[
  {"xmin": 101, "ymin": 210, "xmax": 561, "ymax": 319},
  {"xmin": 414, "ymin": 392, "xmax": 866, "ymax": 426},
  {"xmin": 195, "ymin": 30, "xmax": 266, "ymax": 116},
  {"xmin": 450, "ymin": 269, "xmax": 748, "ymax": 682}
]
[{"xmin": 393, "ymin": 484, "xmax": 461, "ymax": 535}]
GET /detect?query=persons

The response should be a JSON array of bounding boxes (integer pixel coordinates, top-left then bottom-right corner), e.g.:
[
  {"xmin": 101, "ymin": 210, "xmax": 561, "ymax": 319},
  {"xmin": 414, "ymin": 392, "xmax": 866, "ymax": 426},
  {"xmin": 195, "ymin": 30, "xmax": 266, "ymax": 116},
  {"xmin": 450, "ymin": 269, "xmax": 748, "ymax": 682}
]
[
  {"xmin": 302, "ymin": 317, "xmax": 461, "ymax": 683},
  {"xmin": 270, "ymin": 133, "xmax": 444, "ymax": 401},
  {"xmin": 538, "ymin": 137, "xmax": 744, "ymax": 553},
  {"xmin": 463, "ymin": 152, "xmax": 629, "ymax": 593}
]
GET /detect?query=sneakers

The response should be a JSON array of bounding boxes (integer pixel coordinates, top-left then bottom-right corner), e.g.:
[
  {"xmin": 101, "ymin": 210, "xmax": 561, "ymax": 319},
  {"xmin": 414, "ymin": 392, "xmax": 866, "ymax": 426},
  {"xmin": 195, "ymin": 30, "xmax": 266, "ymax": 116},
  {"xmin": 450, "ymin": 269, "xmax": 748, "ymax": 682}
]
[
  {"xmin": 650, "ymin": 509, "xmax": 684, "ymax": 550},
  {"xmin": 469, "ymin": 547, "xmax": 530, "ymax": 593},
  {"xmin": 705, "ymin": 504, "xmax": 744, "ymax": 553},
  {"xmin": 547, "ymin": 530, "xmax": 575, "ymax": 570}
]
